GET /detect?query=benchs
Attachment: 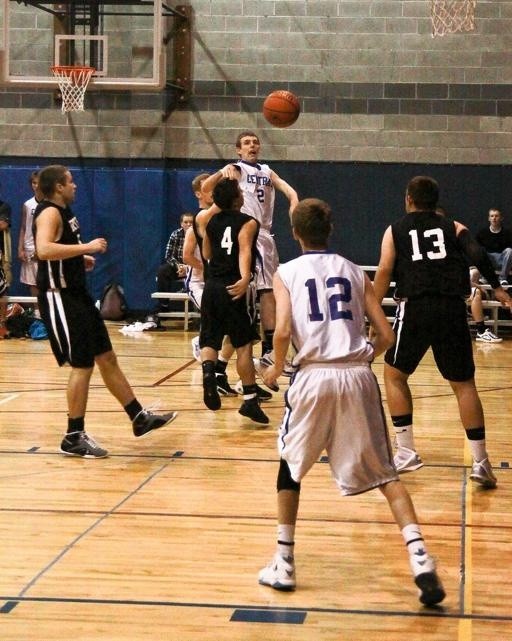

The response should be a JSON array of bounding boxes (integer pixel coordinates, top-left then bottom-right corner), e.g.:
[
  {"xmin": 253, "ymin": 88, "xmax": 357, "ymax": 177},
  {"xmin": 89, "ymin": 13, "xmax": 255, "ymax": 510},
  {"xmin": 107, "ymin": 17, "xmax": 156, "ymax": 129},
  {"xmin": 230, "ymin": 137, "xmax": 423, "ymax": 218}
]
[
  {"xmin": 151, "ymin": 292, "xmax": 512, "ymax": 340},
  {"xmin": 1, "ymin": 294, "xmax": 39, "ymax": 321},
  {"xmin": 155, "ymin": 265, "xmax": 512, "ymax": 326}
]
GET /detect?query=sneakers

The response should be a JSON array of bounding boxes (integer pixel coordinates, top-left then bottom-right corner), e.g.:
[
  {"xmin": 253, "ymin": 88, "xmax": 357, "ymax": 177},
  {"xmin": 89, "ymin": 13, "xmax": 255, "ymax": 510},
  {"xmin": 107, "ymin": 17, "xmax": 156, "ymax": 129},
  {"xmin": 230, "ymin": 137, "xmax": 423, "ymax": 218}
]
[
  {"xmin": 478, "ymin": 277, "xmax": 488, "ymax": 285},
  {"xmin": 409, "ymin": 554, "xmax": 446, "ymax": 605},
  {"xmin": 254, "ymin": 354, "xmax": 280, "ymax": 392},
  {"xmin": 131, "ymin": 407, "xmax": 180, "ymax": 437},
  {"xmin": 214, "ymin": 370, "xmax": 239, "ymax": 398},
  {"xmin": 238, "ymin": 396, "xmax": 269, "ymax": 424},
  {"xmin": 234, "ymin": 379, "xmax": 273, "ymax": 400},
  {"xmin": 475, "ymin": 327, "xmax": 504, "ymax": 343},
  {"xmin": 469, "ymin": 456, "xmax": 498, "ymax": 487},
  {"xmin": 191, "ymin": 336, "xmax": 202, "ymax": 363},
  {"xmin": 261, "ymin": 350, "xmax": 292, "ymax": 377},
  {"xmin": 201, "ymin": 370, "xmax": 222, "ymax": 411},
  {"xmin": 59, "ymin": 430, "xmax": 109, "ymax": 458},
  {"xmin": 0, "ymin": 325, "xmax": 10, "ymax": 339},
  {"xmin": 392, "ymin": 445, "xmax": 424, "ymax": 473},
  {"xmin": 257, "ymin": 553, "xmax": 297, "ymax": 590},
  {"xmin": 499, "ymin": 279, "xmax": 508, "ymax": 285}
]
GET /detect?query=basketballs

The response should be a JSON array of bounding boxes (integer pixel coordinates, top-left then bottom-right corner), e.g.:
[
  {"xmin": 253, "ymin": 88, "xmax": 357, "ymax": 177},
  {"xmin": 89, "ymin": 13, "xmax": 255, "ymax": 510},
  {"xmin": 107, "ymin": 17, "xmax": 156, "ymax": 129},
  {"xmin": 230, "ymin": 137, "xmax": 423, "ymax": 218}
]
[{"xmin": 262, "ymin": 89, "xmax": 301, "ymax": 128}]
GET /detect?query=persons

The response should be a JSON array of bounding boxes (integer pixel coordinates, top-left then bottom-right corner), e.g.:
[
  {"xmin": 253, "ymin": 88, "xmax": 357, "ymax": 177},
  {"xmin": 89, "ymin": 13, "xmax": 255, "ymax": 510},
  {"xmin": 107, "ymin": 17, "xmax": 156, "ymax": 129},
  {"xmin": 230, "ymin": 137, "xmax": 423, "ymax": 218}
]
[
  {"xmin": 0, "ymin": 200, "xmax": 13, "ymax": 339},
  {"xmin": 182, "ymin": 226, "xmax": 205, "ymax": 363},
  {"xmin": 31, "ymin": 165, "xmax": 179, "ymax": 458},
  {"xmin": 257, "ymin": 198, "xmax": 448, "ymax": 607},
  {"xmin": 368, "ymin": 176, "xmax": 512, "ymax": 488},
  {"xmin": 157, "ymin": 211, "xmax": 194, "ymax": 313},
  {"xmin": 477, "ymin": 206, "xmax": 512, "ymax": 285},
  {"xmin": 17, "ymin": 169, "xmax": 44, "ymax": 318},
  {"xmin": 198, "ymin": 178, "xmax": 270, "ymax": 425},
  {"xmin": 467, "ymin": 268, "xmax": 504, "ymax": 344},
  {"xmin": 201, "ymin": 131, "xmax": 300, "ymax": 400},
  {"xmin": 182, "ymin": 174, "xmax": 272, "ymax": 401}
]
